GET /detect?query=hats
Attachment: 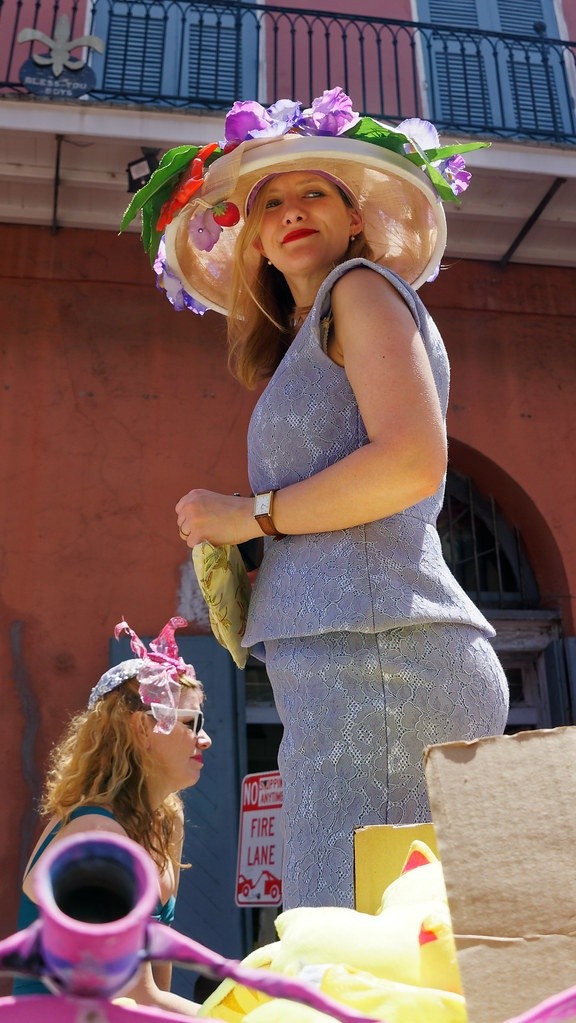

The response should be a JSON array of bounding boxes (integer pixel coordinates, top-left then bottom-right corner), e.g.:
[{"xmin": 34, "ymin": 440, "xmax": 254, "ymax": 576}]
[{"xmin": 120, "ymin": 87, "xmax": 490, "ymax": 319}]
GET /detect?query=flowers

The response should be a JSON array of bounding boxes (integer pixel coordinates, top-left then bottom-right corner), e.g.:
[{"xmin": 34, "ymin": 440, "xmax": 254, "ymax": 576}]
[{"xmin": 116, "ymin": 83, "xmax": 486, "ymax": 313}]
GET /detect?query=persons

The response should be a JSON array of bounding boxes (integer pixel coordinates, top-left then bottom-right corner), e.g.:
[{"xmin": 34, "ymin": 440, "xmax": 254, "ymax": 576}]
[
  {"xmin": 117, "ymin": 88, "xmax": 509, "ymax": 913},
  {"xmin": 14, "ymin": 616, "xmax": 214, "ymax": 1017}
]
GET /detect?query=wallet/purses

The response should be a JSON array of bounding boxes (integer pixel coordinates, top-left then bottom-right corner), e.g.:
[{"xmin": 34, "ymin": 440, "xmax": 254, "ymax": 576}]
[{"xmin": 189, "ymin": 540, "xmax": 252, "ymax": 670}]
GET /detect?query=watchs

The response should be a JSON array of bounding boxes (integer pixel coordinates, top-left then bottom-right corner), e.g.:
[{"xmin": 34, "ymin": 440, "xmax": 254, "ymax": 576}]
[{"xmin": 253, "ymin": 489, "xmax": 286, "ymax": 542}]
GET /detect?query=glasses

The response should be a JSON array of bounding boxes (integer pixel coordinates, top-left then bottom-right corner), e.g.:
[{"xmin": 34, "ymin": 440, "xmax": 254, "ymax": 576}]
[{"xmin": 147, "ymin": 708, "xmax": 205, "ymax": 736}]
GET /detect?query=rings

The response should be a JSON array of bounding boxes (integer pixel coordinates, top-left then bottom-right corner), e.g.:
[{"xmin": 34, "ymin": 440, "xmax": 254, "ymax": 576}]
[{"xmin": 180, "ymin": 530, "xmax": 190, "ymax": 537}]
